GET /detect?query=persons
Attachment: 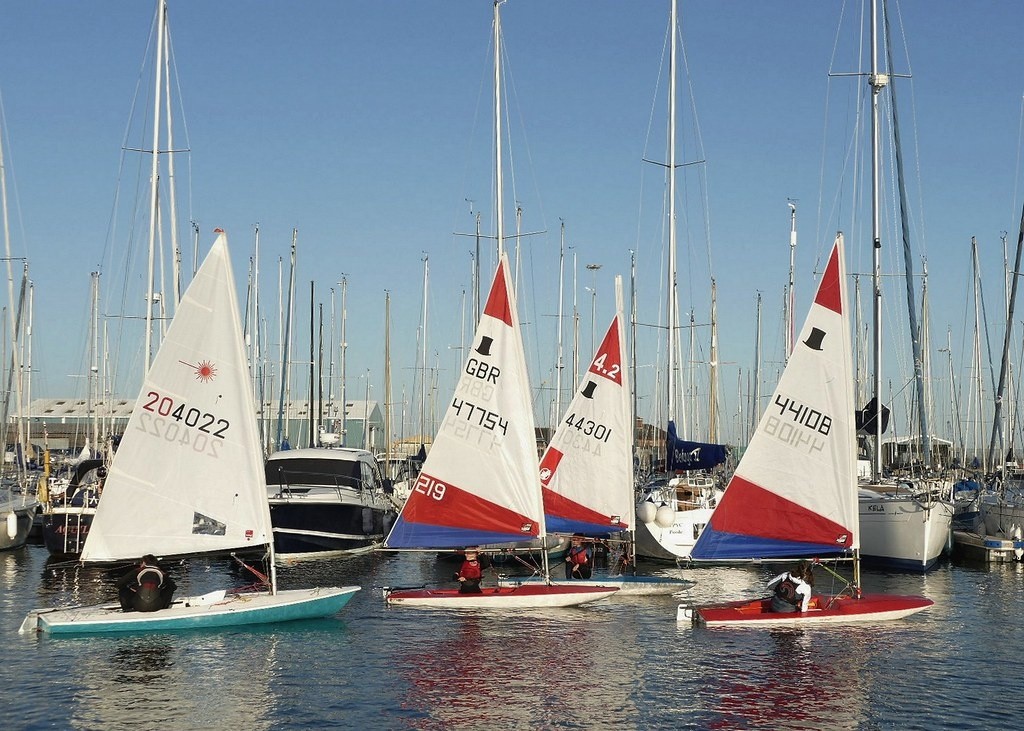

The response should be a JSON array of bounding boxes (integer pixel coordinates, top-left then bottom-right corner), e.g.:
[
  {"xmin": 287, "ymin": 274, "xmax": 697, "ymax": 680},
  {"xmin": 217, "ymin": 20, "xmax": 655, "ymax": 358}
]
[
  {"xmin": 766, "ymin": 560, "xmax": 814, "ymax": 612},
  {"xmin": 565, "ymin": 533, "xmax": 592, "ymax": 579},
  {"xmin": 459, "ymin": 547, "xmax": 490, "ymax": 594},
  {"xmin": 116, "ymin": 554, "xmax": 177, "ymax": 612}
]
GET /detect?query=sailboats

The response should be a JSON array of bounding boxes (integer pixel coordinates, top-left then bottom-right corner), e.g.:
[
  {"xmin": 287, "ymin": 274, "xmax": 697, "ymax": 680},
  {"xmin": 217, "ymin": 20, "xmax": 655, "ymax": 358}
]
[
  {"xmin": 0, "ymin": 0, "xmax": 1024, "ymax": 574},
  {"xmin": 497, "ymin": 274, "xmax": 698, "ymax": 596},
  {"xmin": 382, "ymin": 251, "xmax": 621, "ymax": 609},
  {"xmin": 39, "ymin": 227, "xmax": 361, "ymax": 638},
  {"xmin": 676, "ymin": 231, "xmax": 934, "ymax": 628}
]
